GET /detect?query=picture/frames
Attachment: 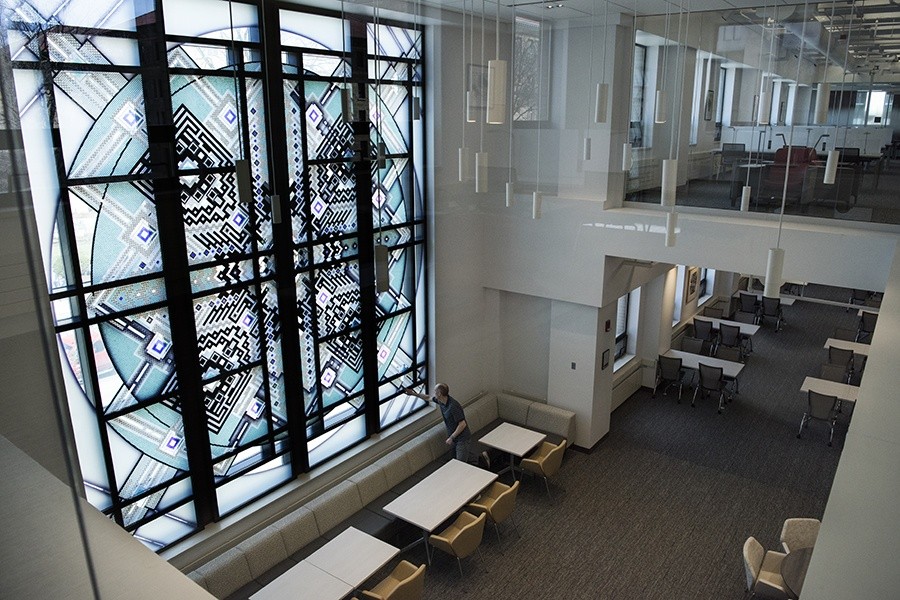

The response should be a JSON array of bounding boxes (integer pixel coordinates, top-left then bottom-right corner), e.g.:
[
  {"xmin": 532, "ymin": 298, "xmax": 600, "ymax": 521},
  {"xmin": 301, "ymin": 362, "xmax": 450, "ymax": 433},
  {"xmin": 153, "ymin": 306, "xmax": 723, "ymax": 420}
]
[
  {"xmin": 705, "ymin": 90, "xmax": 714, "ymax": 120},
  {"xmin": 687, "ymin": 267, "xmax": 698, "ymax": 303}
]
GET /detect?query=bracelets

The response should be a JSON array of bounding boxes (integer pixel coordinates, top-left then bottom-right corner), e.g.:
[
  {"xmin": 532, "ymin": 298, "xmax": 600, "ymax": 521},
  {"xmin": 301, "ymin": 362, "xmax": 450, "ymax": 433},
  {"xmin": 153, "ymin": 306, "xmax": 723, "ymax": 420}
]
[{"xmin": 450, "ymin": 436, "xmax": 455, "ymax": 439}]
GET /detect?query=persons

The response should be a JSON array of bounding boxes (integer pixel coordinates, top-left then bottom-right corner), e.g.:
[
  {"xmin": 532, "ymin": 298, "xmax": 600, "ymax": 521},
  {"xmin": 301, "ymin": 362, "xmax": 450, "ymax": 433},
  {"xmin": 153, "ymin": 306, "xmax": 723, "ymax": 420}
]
[{"xmin": 405, "ymin": 383, "xmax": 490, "ymax": 469}]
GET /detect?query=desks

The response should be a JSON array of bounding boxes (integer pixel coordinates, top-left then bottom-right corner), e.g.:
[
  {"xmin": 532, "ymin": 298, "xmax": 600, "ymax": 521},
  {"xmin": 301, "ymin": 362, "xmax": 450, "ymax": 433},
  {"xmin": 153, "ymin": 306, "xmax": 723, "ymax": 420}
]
[
  {"xmin": 824, "ymin": 338, "xmax": 871, "ymax": 356},
  {"xmin": 780, "ymin": 547, "xmax": 814, "ymax": 599},
  {"xmin": 382, "ymin": 458, "xmax": 498, "ymax": 567},
  {"xmin": 800, "ymin": 376, "xmax": 860, "ymax": 402},
  {"xmin": 732, "ymin": 289, "xmax": 796, "ymax": 305},
  {"xmin": 687, "ymin": 315, "xmax": 760, "ymax": 335},
  {"xmin": 246, "ymin": 559, "xmax": 354, "ymax": 600},
  {"xmin": 663, "ymin": 350, "xmax": 745, "ymax": 379},
  {"xmin": 478, "ymin": 421, "xmax": 547, "ymax": 481},
  {"xmin": 305, "ymin": 526, "xmax": 401, "ymax": 590}
]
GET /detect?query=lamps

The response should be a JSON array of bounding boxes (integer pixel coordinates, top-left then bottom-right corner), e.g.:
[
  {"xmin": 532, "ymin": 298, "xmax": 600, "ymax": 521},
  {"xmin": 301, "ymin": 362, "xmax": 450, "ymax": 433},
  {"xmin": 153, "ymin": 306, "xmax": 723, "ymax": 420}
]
[{"xmin": 230, "ymin": 0, "xmax": 854, "ymax": 299}]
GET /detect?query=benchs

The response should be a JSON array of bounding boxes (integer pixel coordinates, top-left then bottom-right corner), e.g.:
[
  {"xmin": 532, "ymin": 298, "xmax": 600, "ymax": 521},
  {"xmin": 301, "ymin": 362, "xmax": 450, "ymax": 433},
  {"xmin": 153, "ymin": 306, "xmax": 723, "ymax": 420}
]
[
  {"xmin": 499, "ymin": 394, "xmax": 577, "ymax": 448},
  {"xmin": 180, "ymin": 392, "xmax": 499, "ymax": 600}
]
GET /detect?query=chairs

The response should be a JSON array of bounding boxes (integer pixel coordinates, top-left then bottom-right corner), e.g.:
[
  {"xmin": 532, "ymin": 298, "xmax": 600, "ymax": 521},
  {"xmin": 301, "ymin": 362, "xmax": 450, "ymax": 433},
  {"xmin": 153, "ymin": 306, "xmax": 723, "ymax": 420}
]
[
  {"xmin": 651, "ymin": 280, "xmax": 884, "ymax": 447},
  {"xmin": 742, "ymin": 536, "xmax": 787, "ymax": 599},
  {"xmin": 351, "ymin": 440, "xmax": 567, "ymax": 600},
  {"xmin": 717, "ymin": 143, "xmax": 745, "ymax": 182},
  {"xmin": 781, "ymin": 517, "xmax": 820, "ymax": 554},
  {"xmin": 835, "ymin": 147, "xmax": 860, "ymax": 203}
]
